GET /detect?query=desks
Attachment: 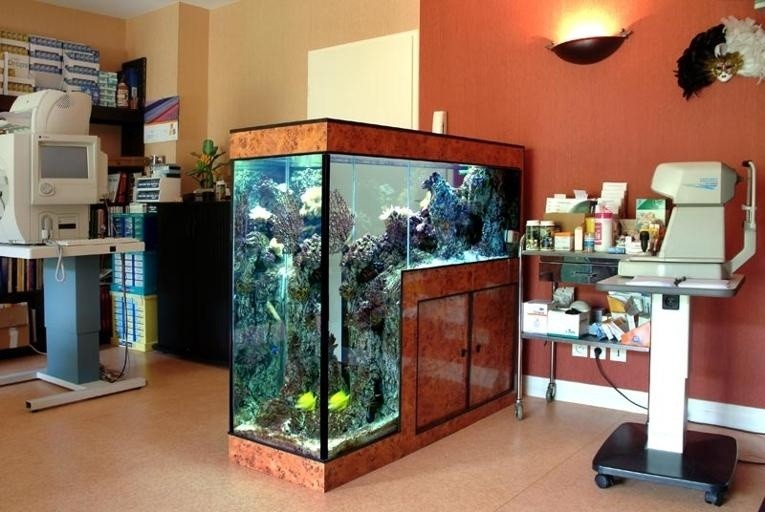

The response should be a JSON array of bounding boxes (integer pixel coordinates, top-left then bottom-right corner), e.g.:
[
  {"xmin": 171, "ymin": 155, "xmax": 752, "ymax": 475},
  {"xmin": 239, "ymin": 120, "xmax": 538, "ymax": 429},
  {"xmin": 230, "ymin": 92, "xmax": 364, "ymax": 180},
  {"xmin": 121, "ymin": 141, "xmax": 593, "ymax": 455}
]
[
  {"xmin": 592, "ymin": 276, "xmax": 743, "ymax": 502},
  {"xmin": 1, "ymin": 243, "xmax": 149, "ymax": 411}
]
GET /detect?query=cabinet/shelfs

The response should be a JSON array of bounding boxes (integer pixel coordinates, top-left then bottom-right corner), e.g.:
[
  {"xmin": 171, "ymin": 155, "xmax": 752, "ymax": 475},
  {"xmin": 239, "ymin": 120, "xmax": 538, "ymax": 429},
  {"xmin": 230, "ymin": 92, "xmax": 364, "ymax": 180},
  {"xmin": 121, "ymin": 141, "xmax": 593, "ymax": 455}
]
[
  {"xmin": 418, "ymin": 284, "xmax": 515, "ymax": 434},
  {"xmin": 1, "ymin": 93, "xmax": 144, "ymax": 361},
  {"xmin": 517, "ymin": 234, "xmax": 650, "ymax": 398},
  {"xmin": 151, "ymin": 201, "xmax": 231, "ymax": 366}
]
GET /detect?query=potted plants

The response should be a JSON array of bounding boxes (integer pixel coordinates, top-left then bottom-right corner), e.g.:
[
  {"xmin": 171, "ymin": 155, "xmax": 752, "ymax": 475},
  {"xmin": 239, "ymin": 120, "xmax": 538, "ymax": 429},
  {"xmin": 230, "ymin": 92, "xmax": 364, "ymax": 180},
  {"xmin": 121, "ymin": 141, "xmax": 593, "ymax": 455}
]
[{"xmin": 186, "ymin": 140, "xmax": 228, "ymax": 202}]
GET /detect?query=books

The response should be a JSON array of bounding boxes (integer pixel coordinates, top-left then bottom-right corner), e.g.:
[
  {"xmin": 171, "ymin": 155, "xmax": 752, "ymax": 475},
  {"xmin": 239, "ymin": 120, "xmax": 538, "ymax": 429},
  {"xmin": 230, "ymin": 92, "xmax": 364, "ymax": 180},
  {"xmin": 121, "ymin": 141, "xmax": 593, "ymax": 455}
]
[
  {"xmin": 0, "ymin": 257, "xmax": 48, "ymax": 351},
  {"xmin": 107, "ymin": 172, "xmax": 131, "ymax": 204}
]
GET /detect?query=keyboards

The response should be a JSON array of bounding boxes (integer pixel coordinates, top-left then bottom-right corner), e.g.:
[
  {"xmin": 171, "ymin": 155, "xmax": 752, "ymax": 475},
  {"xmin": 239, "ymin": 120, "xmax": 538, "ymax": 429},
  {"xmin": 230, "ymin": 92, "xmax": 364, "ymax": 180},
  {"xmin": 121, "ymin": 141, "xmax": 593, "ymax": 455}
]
[{"xmin": 55, "ymin": 237, "xmax": 138, "ymax": 247}]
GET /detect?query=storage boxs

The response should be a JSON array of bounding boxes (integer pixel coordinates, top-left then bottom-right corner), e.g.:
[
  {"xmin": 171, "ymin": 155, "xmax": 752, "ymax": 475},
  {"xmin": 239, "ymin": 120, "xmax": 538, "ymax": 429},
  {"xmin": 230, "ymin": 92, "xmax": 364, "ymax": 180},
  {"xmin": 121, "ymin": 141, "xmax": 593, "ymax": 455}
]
[{"xmin": 0, "ymin": 300, "xmax": 32, "ymax": 349}]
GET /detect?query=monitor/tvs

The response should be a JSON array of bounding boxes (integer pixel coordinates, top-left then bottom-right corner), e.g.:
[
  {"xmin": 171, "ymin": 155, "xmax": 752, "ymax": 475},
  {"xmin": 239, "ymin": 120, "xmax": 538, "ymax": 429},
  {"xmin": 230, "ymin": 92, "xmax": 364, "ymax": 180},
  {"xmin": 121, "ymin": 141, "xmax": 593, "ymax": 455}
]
[{"xmin": 40, "ymin": 146, "xmax": 88, "ymax": 179}]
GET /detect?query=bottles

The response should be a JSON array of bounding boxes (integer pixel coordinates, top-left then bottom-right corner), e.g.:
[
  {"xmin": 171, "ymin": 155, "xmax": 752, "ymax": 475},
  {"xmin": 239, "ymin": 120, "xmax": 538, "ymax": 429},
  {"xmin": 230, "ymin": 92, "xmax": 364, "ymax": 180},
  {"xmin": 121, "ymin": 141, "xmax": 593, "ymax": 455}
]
[
  {"xmin": 215, "ymin": 175, "xmax": 226, "ymax": 202},
  {"xmin": 526, "ymin": 220, "xmax": 555, "ymax": 252},
  {"xmin": 584, "ymin": 212, "xmax": 612, "ymax": 254}
]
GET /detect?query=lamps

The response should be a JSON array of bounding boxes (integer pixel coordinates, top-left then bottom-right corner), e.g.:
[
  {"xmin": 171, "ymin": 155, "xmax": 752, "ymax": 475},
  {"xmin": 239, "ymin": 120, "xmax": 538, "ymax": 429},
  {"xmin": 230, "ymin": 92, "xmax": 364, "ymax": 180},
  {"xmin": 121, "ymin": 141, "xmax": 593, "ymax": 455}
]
[{"xmin": 544, "ymin": 30, "xmax": 633, "ymax": 67}]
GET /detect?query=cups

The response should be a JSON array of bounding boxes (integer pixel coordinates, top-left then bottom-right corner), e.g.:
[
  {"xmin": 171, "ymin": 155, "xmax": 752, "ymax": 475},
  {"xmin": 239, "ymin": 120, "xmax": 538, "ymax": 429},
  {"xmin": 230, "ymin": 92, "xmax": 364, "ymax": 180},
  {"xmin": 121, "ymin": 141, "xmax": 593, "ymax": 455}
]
[{"xmin": 432, "ymin": 111, "xmax": 448, "ymax": 135}]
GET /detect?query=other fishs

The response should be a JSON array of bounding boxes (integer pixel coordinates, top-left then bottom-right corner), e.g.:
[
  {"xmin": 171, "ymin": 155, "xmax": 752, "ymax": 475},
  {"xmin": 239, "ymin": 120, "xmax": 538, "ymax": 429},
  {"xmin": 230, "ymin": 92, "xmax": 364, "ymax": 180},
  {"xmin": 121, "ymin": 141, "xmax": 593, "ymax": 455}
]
[
  {"xmin": 328, "ymin": 390, "xmax": 351, "ymax": 413},
  {"xmin": 294, "ymin": 391, "xmax": 317, "ymax": 413},
  {"xmin": 271, "ymin": 346, "xmax": 280, "ymax": 355}
]
